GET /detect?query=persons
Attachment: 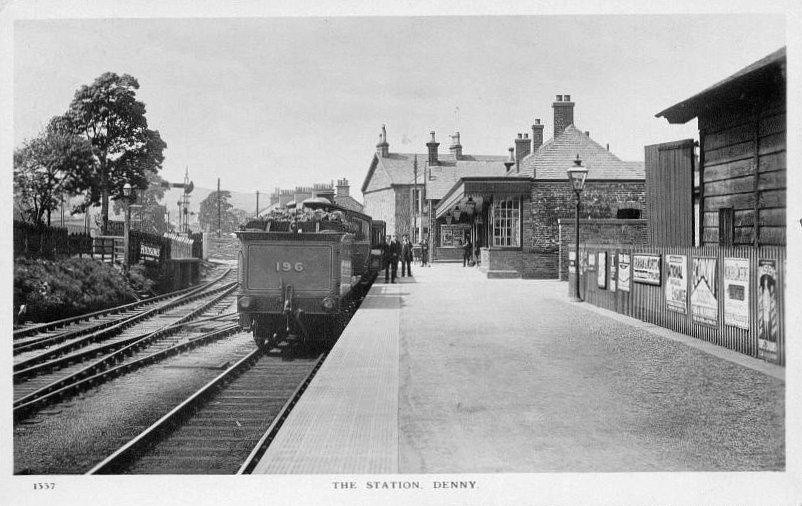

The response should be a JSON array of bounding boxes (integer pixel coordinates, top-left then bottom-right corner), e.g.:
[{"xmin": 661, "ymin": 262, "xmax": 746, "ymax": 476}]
[
  {"xmin": 381, "ymin": 234, "xmax": 397, "ymax": 284},
  {"xmin": 392, "ymin": 234, "xmax": 402, "ymax": 270},
  {"xmin": 462, "ymin": 237, "xmax": 473, "ymax": 266},
  {"xmin": 421, "ymin": 238, "xmax": 429, "ymax": 266},
  {"xmin": 400, "ymin": 233, "xmax": 412, "ymax": 276}
]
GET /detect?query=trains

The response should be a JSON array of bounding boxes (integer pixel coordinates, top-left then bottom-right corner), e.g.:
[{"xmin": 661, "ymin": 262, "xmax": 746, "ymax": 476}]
[{"xmin": 233, "ymin": 192, "xmax": 386, "ymax": 347}]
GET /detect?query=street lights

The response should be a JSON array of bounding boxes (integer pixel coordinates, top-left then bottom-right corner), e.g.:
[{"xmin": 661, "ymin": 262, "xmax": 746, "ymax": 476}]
[
  {"xmin": 120, "ymin": 182, "xmax": 133, "ymax": 267},
  {"xmin": 566, "ymin": 152, "xmax": 588, "ymax": 301}
]
